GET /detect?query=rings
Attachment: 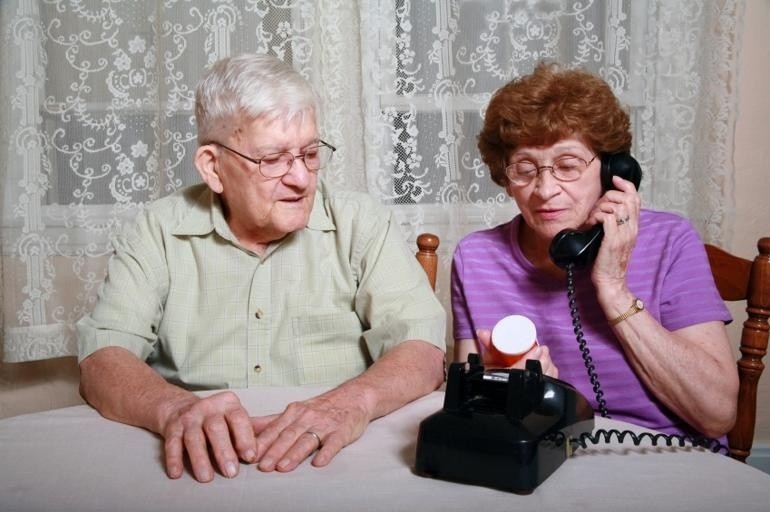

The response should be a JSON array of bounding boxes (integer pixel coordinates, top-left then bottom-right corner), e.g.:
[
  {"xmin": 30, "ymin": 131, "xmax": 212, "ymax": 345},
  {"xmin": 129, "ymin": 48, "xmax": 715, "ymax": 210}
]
[
  {"xmin": 305, "ymin": 431, "xmax": 321, "ymax": 449},
  {"xmin": 616, "ymin": 215, "xmax": 630, "ymax": 225}
]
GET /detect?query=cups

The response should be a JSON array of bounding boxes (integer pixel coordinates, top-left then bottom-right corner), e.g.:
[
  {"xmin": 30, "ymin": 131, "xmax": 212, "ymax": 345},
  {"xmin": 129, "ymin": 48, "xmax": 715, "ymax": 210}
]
[{"xmin": 492, "ymin": 314, "xmax": 539, "ymax": 368}]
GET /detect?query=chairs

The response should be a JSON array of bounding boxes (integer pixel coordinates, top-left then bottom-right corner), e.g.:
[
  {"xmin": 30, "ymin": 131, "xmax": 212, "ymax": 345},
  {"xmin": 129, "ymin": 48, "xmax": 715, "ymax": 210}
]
[
  {"xmin": 414, "ymin": 232, "xmax": 440, "ymax": 293},
  {"xmin": 702, "ymin": 233, "xmax": 770, "ymax": 463}
]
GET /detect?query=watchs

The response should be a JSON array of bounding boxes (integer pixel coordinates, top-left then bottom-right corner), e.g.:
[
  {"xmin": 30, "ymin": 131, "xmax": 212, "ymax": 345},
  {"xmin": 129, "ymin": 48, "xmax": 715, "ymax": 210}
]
[{"xmin": 607, "ymin": 298, "xmax": 644, "ymax": 327}]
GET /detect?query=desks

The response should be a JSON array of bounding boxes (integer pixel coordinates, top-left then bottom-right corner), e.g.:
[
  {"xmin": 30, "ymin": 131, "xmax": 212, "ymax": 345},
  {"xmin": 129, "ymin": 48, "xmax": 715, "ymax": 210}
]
[{"xmin": 2, "ymin": 377, "xmax": 770, "ymax": 512}]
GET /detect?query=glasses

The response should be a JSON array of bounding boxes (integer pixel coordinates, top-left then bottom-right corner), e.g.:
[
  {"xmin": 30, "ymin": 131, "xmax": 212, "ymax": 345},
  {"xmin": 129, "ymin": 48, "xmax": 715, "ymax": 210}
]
[
  {"xmin": 505, "ymin": 153, "xmax": 598, "ymax": 186},
  {"xmin": 209, "ymin": 139, "xmax": 337, "ymax": 179}
]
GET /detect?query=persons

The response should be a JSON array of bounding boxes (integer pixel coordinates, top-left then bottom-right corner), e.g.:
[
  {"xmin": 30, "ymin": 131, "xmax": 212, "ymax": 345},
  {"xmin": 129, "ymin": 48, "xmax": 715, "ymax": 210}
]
[
  {"xmin": 74, "ymin": 53, "xmax": 447, "ymax": 483},
  {"xmin": 450, "ymin": 61, "xmax": 740, "ymax": 456}
]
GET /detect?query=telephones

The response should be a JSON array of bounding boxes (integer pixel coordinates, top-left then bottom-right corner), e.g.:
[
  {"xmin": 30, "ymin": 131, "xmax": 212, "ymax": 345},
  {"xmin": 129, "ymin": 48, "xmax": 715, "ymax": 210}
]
[{"xmin": 548, "ymin": 153, "xmax": 642, "ymax": 270}]
